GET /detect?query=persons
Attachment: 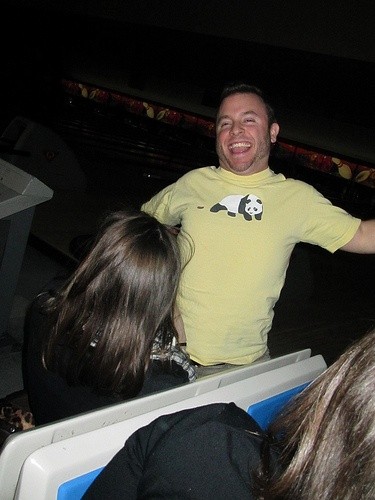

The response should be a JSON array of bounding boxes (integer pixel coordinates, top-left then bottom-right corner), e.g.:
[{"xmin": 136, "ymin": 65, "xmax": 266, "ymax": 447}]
[
  {"xmin": 135, "ymin": 84, "xmax": 375, "ymax": 380},
  {"xmin": 22, "ymin": 212, "xmax": 199, "ymax": 426},
  {"xmin": 80, "ymin": 329, "xmax": 374, "ymax": 500}
]
